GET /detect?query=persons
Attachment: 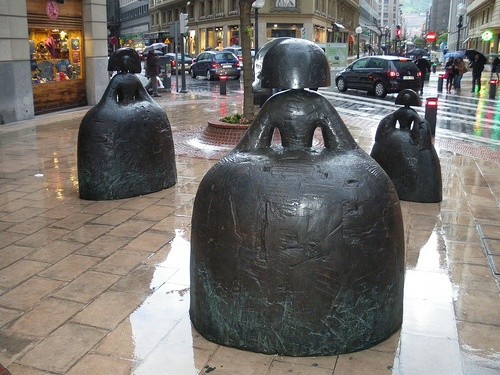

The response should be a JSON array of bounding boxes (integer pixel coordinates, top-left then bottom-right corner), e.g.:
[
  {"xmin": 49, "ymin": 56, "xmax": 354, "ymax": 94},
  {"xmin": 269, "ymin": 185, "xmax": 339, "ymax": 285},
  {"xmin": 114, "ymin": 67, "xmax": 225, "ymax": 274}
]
[
  {"xmin": 412, "ymin": 59, "xmax": 434, "ymax": 95},
  {"xmin": 432, "ymin": 61, "xmax": 438, "ymax": 74},
  {"xmin": 145, "ymin": 50, "xmax": 165, "ymax": 97},
  {"xmin": 445, "ymin": 53, "xmax": 500, "ymax": 99}
]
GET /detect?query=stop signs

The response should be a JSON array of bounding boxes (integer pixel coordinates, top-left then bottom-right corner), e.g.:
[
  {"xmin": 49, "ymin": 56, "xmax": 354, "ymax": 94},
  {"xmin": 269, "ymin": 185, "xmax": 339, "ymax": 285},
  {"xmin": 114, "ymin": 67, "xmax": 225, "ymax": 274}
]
[{"xmin": 426, "ymin": 32, "xmax": 436, "ymax": 43}]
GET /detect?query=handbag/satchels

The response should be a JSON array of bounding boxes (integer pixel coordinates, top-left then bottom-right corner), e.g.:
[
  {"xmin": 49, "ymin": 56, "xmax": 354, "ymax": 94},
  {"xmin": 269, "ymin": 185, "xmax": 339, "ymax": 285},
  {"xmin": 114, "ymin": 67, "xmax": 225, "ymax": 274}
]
[
  {"xmin": 463, "ymin": 66, "xmax": 468, "ymax": 73},
  {"xmin": 444, "ymin": 69, "xmax": 455, "ymax": 79}
]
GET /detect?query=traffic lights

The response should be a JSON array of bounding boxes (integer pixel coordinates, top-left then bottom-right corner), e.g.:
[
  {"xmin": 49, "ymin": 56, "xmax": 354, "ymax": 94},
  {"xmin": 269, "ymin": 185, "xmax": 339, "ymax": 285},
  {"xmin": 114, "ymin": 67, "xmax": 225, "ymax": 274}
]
[{"xmin": 179, "ymin": 13, "xmax": 189, "ymax": 33}]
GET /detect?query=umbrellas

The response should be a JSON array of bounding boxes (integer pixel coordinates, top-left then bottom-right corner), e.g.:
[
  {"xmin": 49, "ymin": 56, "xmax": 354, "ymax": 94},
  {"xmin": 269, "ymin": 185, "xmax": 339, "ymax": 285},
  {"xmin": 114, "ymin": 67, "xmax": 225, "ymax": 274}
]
[
  {"xmin": 483, "ymin": 52, "xmax": 500, "ymax": 59},
  {"xmin": 445, "ymin": 51, "xmax": 464, "ymax": 58},
  {"xmin": 406, "ymin": 46, "xmax": 417, "ymax": 54},
  {"xmin": 464, "ymin": 49, "xmax": 487, "ymax": 67},
  {"xmin": 141, "ymin": 42, "xmax": 169, "ymax": 55},
  {"xmin": 411, "ymin": 47, "xmax": 430, "ymax": 56}
]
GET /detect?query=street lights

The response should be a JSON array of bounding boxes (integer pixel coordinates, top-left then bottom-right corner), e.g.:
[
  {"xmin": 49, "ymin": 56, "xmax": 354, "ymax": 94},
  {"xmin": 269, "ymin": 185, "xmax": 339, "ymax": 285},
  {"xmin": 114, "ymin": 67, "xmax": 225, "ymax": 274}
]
[
  {"xmin": 252, "ymin": 0, "xmax": 266, "ymax": 56},
  {"xmin": 355, "ymin": 27, "xmax": 363, "ymax": 59},
  {"xmin": 456, "ymin": 2, "xmax": 467, "ymax": 52}
]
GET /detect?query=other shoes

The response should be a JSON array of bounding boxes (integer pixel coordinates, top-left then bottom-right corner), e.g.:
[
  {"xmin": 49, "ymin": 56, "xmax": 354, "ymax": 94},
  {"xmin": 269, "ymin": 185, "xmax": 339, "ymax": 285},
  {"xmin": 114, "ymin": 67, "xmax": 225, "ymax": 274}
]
[
  {"xmin": 154, "ymin": 92, "xmax": 161, "ymax": 97},
  {"xmin": 145, "ymin": 86, "xmax": 149, "ymax": 93}
]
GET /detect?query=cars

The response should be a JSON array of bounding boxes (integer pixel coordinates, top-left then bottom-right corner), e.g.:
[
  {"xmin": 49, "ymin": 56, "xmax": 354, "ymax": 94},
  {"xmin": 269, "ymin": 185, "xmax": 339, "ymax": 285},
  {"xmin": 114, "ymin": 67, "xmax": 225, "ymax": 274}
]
[
  {"xmin": 334, "ymin": 55, "xmax": 422, "ymax": 98},
  {"xmin": 189, "ymin": 50, "xmax": 241, "ymax": 80},
  {"xmin": 166, "ymin": 53, "xmax": 192, "ymax": 74},
  {"xmin": 224, "ymin": 48, "xmax": 255, "ymax": 69}
]
